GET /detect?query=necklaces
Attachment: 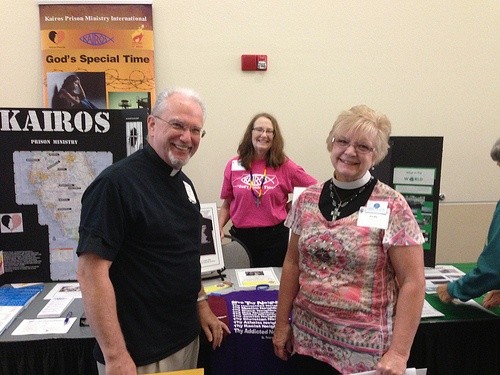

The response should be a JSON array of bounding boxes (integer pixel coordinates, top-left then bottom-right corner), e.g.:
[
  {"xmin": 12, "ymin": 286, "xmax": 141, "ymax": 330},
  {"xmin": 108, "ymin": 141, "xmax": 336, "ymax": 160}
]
[
  {"xmin": 248, "ymin": 160, "xmax": 268, "ymax": 208},
  {"xmin": 327, "ymin": 177, "xmax": 372, "ymax": 221}
]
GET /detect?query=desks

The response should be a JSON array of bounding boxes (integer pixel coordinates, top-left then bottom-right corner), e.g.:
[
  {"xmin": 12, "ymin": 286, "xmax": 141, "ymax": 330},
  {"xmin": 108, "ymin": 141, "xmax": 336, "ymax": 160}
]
[{"xmin": 0, "ymin": 263, "xmax": 500, "ymax": 375}]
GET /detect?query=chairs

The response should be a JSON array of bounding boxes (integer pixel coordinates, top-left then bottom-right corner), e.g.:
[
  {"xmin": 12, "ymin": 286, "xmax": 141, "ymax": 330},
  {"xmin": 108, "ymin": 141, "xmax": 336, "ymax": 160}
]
[{"xmin": 220, "ymin": 234, "xmax": 252, "ymax": 269}]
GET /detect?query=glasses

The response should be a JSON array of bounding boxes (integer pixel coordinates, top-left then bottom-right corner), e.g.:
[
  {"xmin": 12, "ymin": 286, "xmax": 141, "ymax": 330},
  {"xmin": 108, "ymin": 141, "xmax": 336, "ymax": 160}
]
[
  {"xmin": 253, "ymin": 128, "xmax": 273, "ymax": 135},
  {"xmin": 154, "ymin": 115, "xmax": 207, "ymax": 139},
  {"xmin": 335, "ymin": 137, "xmax": 375, "ymax": 155}
]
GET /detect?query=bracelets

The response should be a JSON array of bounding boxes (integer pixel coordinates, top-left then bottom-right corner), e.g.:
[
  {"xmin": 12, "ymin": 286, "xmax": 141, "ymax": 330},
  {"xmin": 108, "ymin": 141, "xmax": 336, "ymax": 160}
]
[{"xmin": 196, "ymin": 294, "xmax": 208, "ymax": 303}]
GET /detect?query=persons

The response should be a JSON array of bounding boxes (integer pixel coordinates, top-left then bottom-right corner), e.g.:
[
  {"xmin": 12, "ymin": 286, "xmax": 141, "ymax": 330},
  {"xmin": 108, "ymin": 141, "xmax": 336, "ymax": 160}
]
[
  {"xmin": 76, "ymin": 89, "xmax": 231, "ymax": 375},
  {"xmin": 271, "ymin": 106, "xmax": 426, "ymax": 375},
  {"xmin": 218, "ymin": 112, "xmax": 318, "ymax": 268},
  {"xmin": 434, "ymin": 137, "xmax": 500, "ymax": 307}
]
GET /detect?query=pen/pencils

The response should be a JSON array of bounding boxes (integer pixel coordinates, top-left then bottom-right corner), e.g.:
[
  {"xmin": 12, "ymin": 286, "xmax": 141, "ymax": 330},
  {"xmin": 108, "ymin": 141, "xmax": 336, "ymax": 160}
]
[{"xmin": 63, "ymin": 311, "xmax": 73, "ymax": 326}]
